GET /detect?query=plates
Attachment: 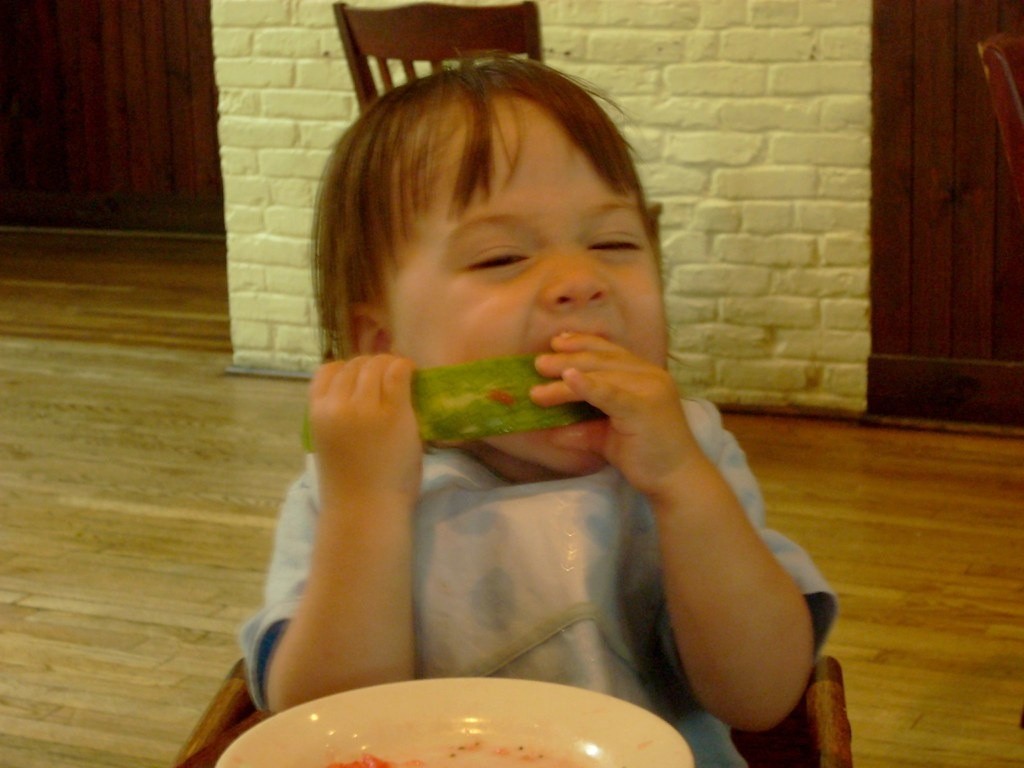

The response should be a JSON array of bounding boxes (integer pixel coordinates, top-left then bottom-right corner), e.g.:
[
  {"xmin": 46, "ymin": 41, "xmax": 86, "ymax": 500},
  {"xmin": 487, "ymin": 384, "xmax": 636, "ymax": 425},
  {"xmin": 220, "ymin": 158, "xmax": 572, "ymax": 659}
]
[{"xmin": 211, "ymin": 676, "xmax": 696, "ymax": 768}]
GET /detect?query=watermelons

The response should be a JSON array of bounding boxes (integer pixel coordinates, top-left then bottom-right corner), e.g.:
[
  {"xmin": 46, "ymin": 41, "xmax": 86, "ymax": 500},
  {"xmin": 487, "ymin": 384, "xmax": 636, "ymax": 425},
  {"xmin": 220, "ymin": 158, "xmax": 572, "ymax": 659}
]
[{"xmin": 299, "ymin": 353, "xmax": 603, "ymax": 461}]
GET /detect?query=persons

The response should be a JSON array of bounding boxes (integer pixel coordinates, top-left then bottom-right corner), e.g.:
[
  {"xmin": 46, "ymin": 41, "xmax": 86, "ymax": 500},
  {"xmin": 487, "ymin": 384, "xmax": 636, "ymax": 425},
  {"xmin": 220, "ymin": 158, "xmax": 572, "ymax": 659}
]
[{"xmin": 241, "ymin": 49, "xmax": 838, "ymax": 767}]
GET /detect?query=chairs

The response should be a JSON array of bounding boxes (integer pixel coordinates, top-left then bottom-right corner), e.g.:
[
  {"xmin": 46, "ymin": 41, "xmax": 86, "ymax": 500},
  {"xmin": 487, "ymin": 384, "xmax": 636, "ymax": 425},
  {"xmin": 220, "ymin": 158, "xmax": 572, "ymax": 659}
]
[
  {"xmin": 172, "ymin": 652, "xmax": 856, "ymax": 768},
  {"xmin": 332, "ymin": 0, "xmax": 542, "ymax": 113}
]
